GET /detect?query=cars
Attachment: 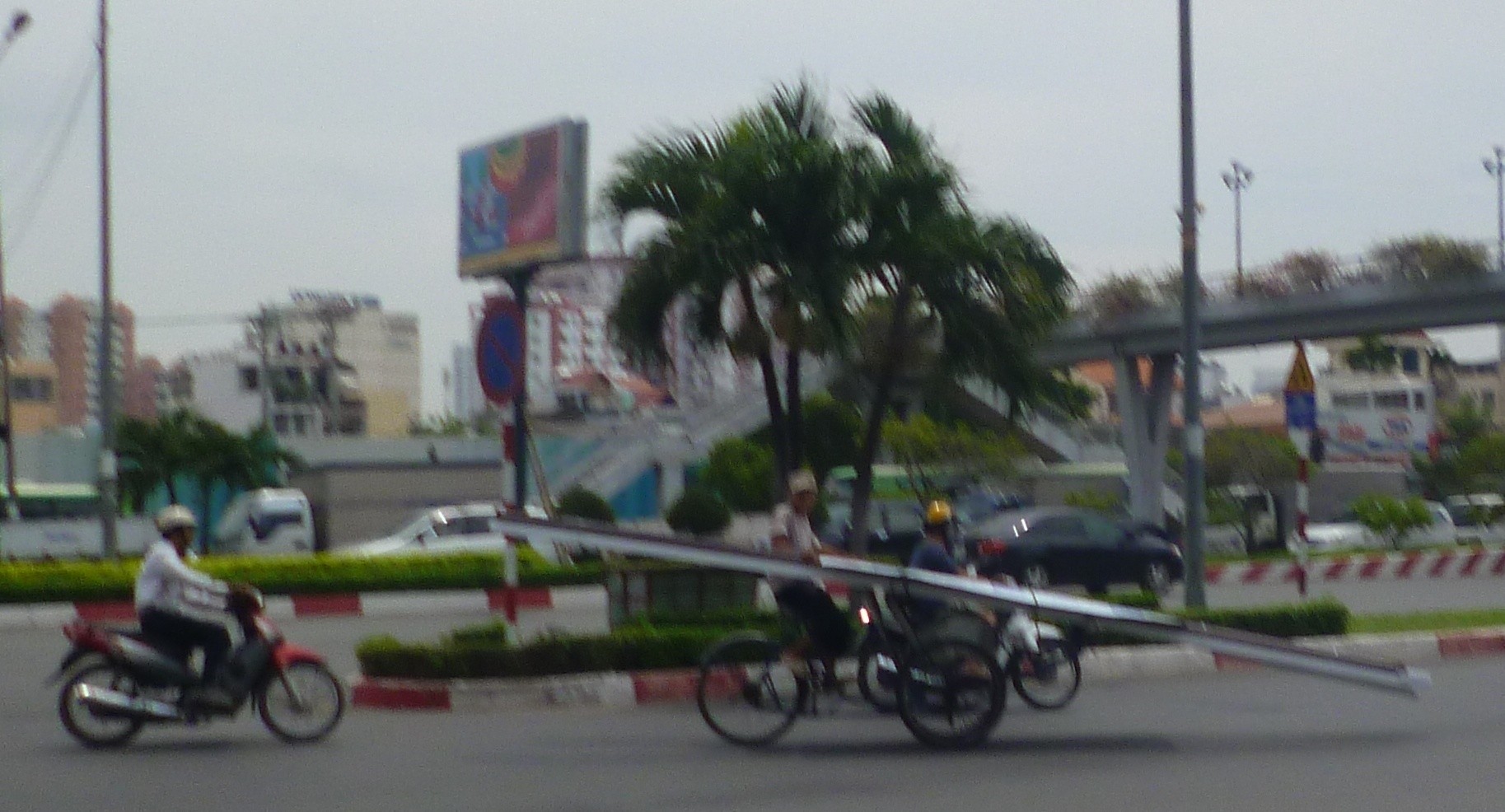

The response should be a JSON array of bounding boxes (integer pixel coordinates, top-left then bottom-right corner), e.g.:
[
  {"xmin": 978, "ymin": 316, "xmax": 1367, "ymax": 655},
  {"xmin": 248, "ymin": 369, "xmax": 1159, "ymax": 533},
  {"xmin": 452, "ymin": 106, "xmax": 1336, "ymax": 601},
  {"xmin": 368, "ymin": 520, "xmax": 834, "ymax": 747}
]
[
  {"xmin": 322, "ymin": 501, "xmax": 559, "ymax": 561},
  {"xmin": 1304, "ymin": 496, "xmax": 1457, "ymax": 551},
  {"xmin": 957, "ymin": 504, "xmax": 1187, "ymax": 595},
  {"xmin": 1445, "ymin": 495, "xmax": 1504, "ymax": 546}
]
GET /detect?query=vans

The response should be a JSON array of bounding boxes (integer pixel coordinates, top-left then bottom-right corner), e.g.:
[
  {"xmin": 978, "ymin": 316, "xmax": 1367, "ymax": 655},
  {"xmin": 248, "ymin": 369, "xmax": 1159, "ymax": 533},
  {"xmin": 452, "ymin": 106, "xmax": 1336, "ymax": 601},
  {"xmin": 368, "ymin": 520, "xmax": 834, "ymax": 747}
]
[{"xmin": 1197, "ymin": 477, "xmax": 1309, "ymax": 557}]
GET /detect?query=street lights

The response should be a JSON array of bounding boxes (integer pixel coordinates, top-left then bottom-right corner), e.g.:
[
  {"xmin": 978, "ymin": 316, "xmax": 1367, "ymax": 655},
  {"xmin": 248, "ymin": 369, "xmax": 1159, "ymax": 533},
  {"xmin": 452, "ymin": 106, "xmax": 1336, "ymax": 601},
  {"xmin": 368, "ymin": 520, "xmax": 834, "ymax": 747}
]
[
  {"xmin": 1220, "ymin": 159, "xmax": 1254, "ymax": 304},
  {"xmin": 1480, "ymin": 146, "xmax": 1504, "ymax": 277}
]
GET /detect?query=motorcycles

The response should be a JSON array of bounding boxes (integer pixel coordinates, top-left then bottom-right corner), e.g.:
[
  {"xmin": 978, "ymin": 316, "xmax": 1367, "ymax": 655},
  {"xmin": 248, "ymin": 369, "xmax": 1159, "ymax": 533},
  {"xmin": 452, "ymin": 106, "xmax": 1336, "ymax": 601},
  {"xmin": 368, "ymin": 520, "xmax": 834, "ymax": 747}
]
[{"xmin": 45, "ymin": 580, "xmax": 344, "ymax": 754}]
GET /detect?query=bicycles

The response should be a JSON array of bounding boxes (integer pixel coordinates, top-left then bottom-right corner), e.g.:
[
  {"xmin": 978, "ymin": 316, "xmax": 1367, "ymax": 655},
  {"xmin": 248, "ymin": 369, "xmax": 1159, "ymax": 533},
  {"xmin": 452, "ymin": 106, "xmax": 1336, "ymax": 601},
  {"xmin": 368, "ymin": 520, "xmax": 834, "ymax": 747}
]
[{"xmin": 693, "ymin": 584, "xmax": 1010, "ymax": 751}]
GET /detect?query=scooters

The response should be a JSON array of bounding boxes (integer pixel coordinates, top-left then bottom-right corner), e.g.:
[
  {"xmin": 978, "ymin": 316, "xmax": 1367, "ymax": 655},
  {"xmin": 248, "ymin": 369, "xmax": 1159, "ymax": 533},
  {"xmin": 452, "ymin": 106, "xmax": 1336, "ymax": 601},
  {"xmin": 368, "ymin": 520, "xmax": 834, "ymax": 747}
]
[{"xmin": 859, "ymin": 566, "xmax": 1082, "ymax": 717}]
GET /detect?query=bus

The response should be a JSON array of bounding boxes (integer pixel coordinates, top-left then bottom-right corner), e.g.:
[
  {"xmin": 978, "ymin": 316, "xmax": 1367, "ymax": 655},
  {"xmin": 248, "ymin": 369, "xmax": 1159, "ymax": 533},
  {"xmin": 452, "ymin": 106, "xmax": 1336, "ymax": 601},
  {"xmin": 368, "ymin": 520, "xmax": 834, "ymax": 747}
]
[{"xmin": 802, "ymin": 460, "xmax": 1128, "ymax": 546}]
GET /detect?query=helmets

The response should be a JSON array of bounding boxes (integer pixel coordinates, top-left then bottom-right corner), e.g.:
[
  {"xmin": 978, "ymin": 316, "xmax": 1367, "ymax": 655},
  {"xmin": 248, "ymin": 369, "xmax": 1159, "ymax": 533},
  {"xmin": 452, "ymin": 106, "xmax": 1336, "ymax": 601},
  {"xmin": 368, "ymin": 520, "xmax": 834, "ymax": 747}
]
[
  {"xmin": 155, "ymin": 505, "xmax": 197, "ymax": 530},
  {"xmin": 925, "ymin": 501, "xmax": 951, "ymax": 525},
  {"xmin": 790, "ymin": 469, "xmax": 818, "ymax": 495}
]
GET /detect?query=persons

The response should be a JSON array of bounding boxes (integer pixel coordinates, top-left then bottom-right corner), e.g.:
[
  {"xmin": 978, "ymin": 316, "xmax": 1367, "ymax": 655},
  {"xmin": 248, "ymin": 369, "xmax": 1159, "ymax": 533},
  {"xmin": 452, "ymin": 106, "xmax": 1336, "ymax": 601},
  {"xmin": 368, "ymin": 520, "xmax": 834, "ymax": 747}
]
[
  {"xmin": 906, "ymin": 501, "xmax": 1010, "ymax": 680},
  {"xmin": 767, "ymin": 469, "xmax": 852, "ymax": 691},
  {"xmin": 136, "ymin": 504, "xmax": 247, "ymax": 708}
]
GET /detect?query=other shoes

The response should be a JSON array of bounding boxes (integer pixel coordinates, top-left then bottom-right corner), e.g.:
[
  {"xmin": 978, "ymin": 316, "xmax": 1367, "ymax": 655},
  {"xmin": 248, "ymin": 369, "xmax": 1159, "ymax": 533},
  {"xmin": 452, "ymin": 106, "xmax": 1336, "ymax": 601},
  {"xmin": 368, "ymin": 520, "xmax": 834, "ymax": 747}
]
[{"xmin": 200, "ymin": 687, "xmax": 232, "ymax": 708}]
